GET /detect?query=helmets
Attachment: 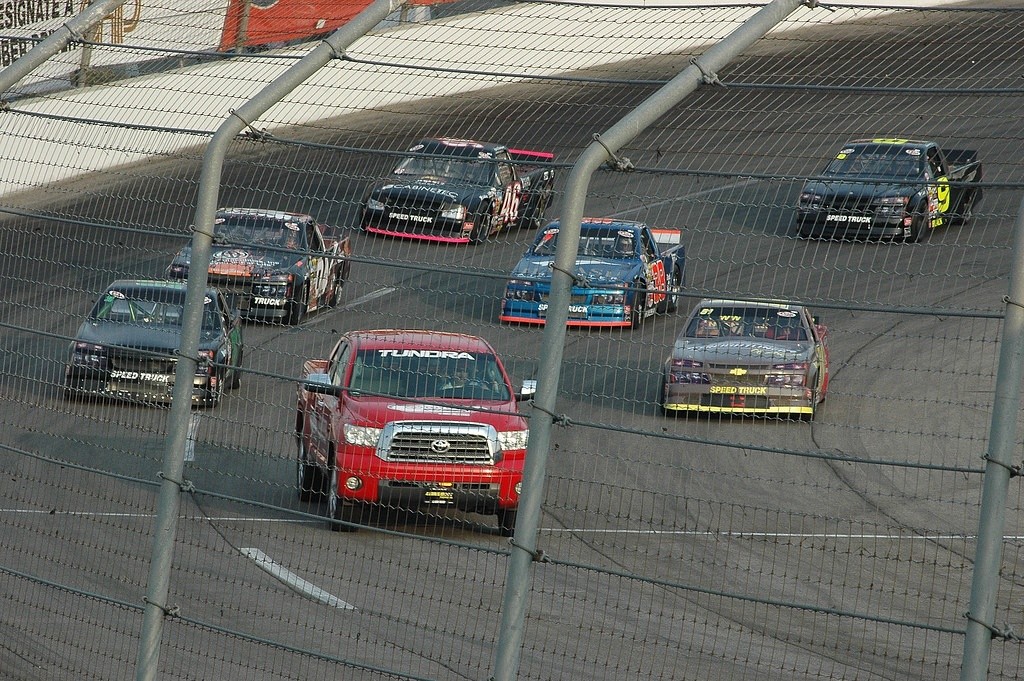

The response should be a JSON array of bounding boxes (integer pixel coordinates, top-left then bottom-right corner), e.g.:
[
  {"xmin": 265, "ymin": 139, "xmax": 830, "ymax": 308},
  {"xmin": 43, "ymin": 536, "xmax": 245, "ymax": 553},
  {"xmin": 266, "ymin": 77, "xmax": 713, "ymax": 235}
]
[
  {"xmin": 614, "ymin": 236, "xmax": 632, "ymax": 254},
  {"xmin": 283, "ymin": 227, "xmax": 297, "ymax": 246}
]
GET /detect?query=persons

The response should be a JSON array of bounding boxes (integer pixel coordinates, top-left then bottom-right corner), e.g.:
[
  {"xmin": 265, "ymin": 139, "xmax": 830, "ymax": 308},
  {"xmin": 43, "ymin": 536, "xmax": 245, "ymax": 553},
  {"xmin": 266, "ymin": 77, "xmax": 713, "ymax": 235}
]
[
  {"xmin": 778, "ymin": 320, "xmax": 792, "ymax": 335},
  {"xmin": 613, "ymin": 236, "xmax": 633, "ymax": 255},
  {"xmin": 466, "ymin": 162, "xmax": 481, "ymax": 179},
  {"xmin": 441, "ymin": 362, "xmax": 499, "ymax": 392},
  {"xmin": 910, "ymin": 156, "xmax": 919, "ymax": 173},
  {"xmin": 276, "ymin": 228, "xmax": 296, "ymax": 248}
]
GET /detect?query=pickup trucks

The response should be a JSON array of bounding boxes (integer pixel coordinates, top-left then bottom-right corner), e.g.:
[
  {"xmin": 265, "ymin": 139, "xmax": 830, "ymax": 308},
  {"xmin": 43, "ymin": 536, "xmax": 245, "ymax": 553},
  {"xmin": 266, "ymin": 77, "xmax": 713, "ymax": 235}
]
[
  {"xmin": 357, "ymin": 136, "xmax": 556, "ymax": 245},
  {"xmin": 660, "ymin": 297, "xmax": 829, "ymax": 421},
  {"xmin": 797, "ymin": 137, "xmax": 984, "ymax": 245},
  {"xmin": 497, "ymin": 215, "xmax": 686, "ymax": 330},
  {"xmin": 291, "ymin": 327, "xmax": 539, "ymax": 537},
  {"xmin": 61, "ymin": 279, "xmax": 245, "ymax": 408},
  {"xmin": 163, "ymin": 206, "xmax": 352, "ymax": 326}
]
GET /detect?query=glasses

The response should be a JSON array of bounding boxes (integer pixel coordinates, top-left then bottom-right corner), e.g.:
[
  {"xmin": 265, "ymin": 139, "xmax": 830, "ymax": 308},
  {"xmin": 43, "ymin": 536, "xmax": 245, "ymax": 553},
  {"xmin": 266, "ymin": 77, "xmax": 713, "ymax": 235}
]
[{"xmin": 455, "ymin": 367, "xmax": 470, "ymax": 373}]
